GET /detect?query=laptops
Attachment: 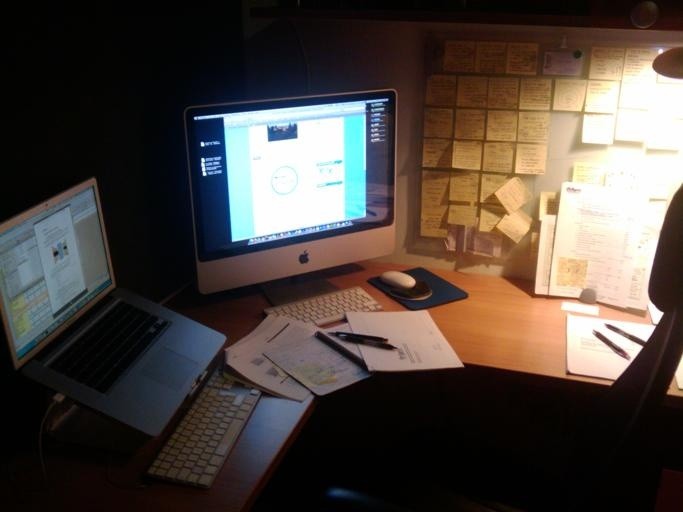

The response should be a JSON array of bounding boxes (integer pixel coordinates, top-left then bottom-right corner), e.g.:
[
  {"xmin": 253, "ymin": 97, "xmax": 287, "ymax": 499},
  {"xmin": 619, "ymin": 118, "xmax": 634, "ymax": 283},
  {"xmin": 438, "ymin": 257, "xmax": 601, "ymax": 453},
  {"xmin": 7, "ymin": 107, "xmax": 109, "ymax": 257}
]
[{"xmin": 0, "ymin": 177, "xmax": 228, "ymax": 436}]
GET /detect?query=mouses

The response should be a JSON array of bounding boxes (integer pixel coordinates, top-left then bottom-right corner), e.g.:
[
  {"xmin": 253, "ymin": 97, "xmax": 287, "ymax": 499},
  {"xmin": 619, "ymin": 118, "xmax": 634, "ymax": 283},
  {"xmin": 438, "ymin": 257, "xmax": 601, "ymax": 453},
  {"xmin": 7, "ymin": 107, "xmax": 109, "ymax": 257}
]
[{"xmin": 381, "ymin": 271, "xmax": 416, "ymax": 289}]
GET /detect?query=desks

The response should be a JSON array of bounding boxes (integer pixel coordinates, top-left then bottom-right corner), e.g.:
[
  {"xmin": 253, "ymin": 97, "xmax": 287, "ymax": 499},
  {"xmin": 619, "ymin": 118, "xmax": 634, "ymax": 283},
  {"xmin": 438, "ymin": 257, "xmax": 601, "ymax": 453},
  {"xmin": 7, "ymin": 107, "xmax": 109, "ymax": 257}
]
[{"xmin": 29, "ymin": 262, "xmax": 683, "ymax": 512}]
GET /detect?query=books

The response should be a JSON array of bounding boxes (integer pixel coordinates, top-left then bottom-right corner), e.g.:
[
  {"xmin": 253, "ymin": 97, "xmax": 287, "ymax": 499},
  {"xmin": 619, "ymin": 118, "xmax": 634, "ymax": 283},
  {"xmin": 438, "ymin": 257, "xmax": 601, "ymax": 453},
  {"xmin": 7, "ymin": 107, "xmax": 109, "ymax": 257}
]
[
  {"xmin": 222, "ymin": 314, "xmax": 326, "ymax": 402},
  {"xmin": 565, "ymin": 312, "xmax": 656, "ymax": 382},
  {"xmin": 344, "ymin": 309, "xmax": 465, "ymax": 373},
  {"xmin": 261, "ymin": 322, "xmax": 377, "ymax": 397}
]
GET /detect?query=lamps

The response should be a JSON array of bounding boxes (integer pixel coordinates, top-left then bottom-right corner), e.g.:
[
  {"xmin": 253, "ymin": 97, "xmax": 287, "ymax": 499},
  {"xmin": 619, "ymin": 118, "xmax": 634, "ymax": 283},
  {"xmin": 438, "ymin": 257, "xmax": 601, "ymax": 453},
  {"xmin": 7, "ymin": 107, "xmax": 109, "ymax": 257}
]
[{"xmin": 612, "ymin": 46, "xmax": 683, "ymax": 398}]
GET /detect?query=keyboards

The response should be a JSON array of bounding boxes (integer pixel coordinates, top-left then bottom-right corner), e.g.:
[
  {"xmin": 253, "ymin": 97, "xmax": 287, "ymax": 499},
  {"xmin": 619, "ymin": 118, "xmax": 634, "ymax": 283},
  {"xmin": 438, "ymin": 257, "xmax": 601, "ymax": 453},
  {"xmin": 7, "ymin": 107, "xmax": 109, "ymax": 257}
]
[
  {"xmin": 264, "ymin": 286, "xmax": 384, "ymax": 326},
  {"xmin": 148, "ymin": 365, "xmax": 261, "ymax": 488}
]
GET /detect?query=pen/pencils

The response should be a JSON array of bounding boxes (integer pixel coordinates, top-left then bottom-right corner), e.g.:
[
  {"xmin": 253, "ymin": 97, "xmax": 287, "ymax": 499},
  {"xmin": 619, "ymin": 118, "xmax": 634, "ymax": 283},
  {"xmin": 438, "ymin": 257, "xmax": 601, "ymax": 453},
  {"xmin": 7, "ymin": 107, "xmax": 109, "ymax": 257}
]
[
  {"xmin": 315, "ymin": 330, "xmax": 399, "ymax": 371},
  {"xmin": 592, "ymin": 322, "xmax": 647, "ymax": 360}
]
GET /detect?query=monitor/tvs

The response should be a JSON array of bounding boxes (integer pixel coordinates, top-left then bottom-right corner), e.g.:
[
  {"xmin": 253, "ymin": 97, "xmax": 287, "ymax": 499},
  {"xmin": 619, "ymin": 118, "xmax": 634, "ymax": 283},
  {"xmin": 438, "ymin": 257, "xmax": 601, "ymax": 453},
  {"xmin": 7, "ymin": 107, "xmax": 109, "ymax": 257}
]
[{"xmin": 184, "ymin": 88, "xmax": 398, "ymax": 306}]
